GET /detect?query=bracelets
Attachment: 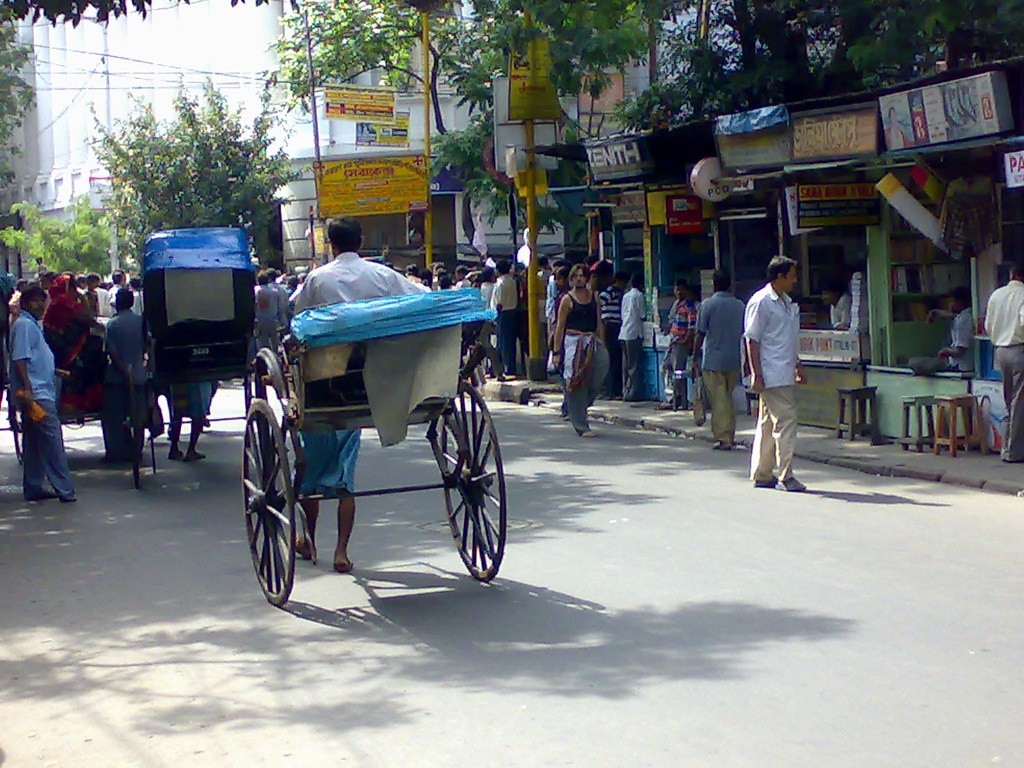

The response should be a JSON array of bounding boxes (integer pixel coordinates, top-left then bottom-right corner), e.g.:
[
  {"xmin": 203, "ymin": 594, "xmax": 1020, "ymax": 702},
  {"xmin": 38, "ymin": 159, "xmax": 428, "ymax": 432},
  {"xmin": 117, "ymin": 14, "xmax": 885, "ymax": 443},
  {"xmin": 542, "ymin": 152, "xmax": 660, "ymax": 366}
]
[{"xmin": 552, "ymin": 351, "xmax": 560, "ymax": 356}]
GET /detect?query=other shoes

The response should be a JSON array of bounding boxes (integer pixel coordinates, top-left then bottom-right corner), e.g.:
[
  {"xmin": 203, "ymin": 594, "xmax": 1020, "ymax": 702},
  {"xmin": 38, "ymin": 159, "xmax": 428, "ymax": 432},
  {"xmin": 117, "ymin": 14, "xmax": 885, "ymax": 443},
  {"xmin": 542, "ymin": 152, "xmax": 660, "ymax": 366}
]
[
  {"xmin": 183, "ymin": 450, "xmax": 207, "ymax": 461},
  {"xmin": 169, "ymin": 452, "xmax": 183, "ymax": 460},
  {"xmin": 24, "ymin": 491, "xmax": 56, "ymax": 501},
  {"xmin": 673, "ymin": 394, "xmax": 682, "ymax": 411},
  {"xmin": 502, "ymin": 372, "xmax": 517, "ymax": 380},
  {"xmin": 1003, "ymin": 457, "xmax": 1024, "ymax": 463},
  {"xmin": 756, "ymin": 475, "xmax": 779, "ymax": 488},
  {"xmin": 712, "ymin": 440, "xmax": 732, "ymax": 449},
  {"xmin": 775, "ymin": 477, "xmax": 806, "ymax": 492},
  {"xmin": 332, "ymin": 558, "xmax": 353, "ymax": 572},
  {"xmin": 580, "ymin": 430, "xmax": 597, "ymax": 437},
  {"xmin": 655, "ymin": 402, "xmax": 673, "ymax": 410},
  {"xmin": 294, "ymin": 533, "xmax": 317, "ymax": 559},
  {"xmin": 57, "ymin": 491, "xmax": 79, "ymax": 502},
  {"xmin": 562, "ymin": 412, "xmax": 570, "ymax": 421}
]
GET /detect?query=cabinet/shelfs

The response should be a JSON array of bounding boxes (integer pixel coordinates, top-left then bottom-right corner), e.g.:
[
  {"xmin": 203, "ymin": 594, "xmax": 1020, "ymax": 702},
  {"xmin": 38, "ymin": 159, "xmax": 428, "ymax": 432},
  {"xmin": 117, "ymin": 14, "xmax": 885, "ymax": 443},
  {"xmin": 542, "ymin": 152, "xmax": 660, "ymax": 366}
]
[{"xmin": 866, "ymin": 226, "xmax": 980, "ymax": 363}]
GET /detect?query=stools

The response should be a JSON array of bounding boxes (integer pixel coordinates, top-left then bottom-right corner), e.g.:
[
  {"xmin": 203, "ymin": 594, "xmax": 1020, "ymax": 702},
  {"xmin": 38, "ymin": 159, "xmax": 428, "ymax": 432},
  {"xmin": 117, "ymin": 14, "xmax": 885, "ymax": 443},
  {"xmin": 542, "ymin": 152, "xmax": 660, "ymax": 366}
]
[
  {"xmin": 836, "ymin": 385, "xmax": 878, "ymax": 441},
  {"xmin": 933, "ymin": 394, "xmax": 989, "ymax": 457},
  {"xmin": 898, "ymin": 396, "xmax": 932, "ymax": 452},
  {"xmin": 745, "ymin": 387, "xmax": 765, "ymax": 416},
  {"xmin": 671, "ymin": 368, "xmax": 709, "ymax": 410}
]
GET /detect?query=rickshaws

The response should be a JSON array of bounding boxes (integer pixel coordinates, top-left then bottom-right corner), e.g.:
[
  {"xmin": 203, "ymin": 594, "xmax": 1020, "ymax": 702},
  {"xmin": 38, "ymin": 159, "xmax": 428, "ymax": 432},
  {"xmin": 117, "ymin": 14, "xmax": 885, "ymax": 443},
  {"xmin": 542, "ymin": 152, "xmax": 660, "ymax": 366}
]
[
  {"xmin": 141, "ymin": 226, "xmax": 295, "ymax": 474},
  {"xmin": 241, "ymin": 286, "xmax": 508, "ymax": 606},
  {"xmin": 5, "ymin": 320, "xmax": 141, "ymax": 467}
]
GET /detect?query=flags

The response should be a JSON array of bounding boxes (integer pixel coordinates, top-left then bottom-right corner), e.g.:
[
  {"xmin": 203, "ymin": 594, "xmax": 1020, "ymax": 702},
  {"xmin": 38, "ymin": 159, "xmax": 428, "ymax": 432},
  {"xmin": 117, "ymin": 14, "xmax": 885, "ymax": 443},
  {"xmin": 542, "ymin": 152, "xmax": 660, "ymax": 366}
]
[{"xmin": 471, "ymin": 207, "xmax": 488, "ymax": 255}]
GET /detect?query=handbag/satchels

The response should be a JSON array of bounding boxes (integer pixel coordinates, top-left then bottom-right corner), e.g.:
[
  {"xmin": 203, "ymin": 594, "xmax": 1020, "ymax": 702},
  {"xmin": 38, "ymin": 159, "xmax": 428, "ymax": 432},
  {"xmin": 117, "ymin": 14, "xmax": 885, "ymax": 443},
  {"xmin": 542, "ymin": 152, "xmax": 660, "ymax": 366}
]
[
  {"xmin": 908, "ymin": 356, "xmax": 940, "ymax": 376},
  {"xmin": 694, "ymin": 399, "xmax": 706, "ymax": 426}
]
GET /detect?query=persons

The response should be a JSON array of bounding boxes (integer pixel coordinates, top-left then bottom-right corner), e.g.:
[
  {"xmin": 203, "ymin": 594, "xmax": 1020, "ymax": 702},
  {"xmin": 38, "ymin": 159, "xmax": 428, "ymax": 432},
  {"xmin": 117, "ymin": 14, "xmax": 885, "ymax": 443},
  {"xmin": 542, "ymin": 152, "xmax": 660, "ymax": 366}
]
[
  {"xmin": 254, "ymin": 249, "xmax": 310, "ymax": 372},
  {"xmin": 293, "ymin": 430, "xmax": 361, "ymax": 572},
  {"xmin": 980, "ymin": 395, "xmax": 1002, "ymax": 448},
  {"xmin": 883, "ymin": 107, "xmax": 914, "ymax": 149},
  {"xmin": 984, "ymin": 255, "xmax": 1024, "ymax": 465},
  {"xmin": 587, "ymin": 259, "xmax": 701, "ymax": 410},
  {"xmin": 551, "ymin": 264, "xmax": 601, "ymax": 438},
  {"xmin": 820, "ymin": 282, "xmax": 851, "ymax": 330},
  {"xmin": 744, "ymin": 255, "xmax": 808, "ymax": 492},
  {"xmin": 294, "ymin": 216, "xmax": 428, "ymax": 313},
  {"xmin": 0, "ymin": 254, "xmax": 219, "ymax": 501},
  {"xmin": 909, "ymin": 285, "xmax": 975, "ymax": 373},
  {"xmin": 386, "ymin": 256, "xmax": 574, "ymax": 382},
  {"xmin": 692, "ymin": 270, "xmax": 751, "ymax": 451}
]
